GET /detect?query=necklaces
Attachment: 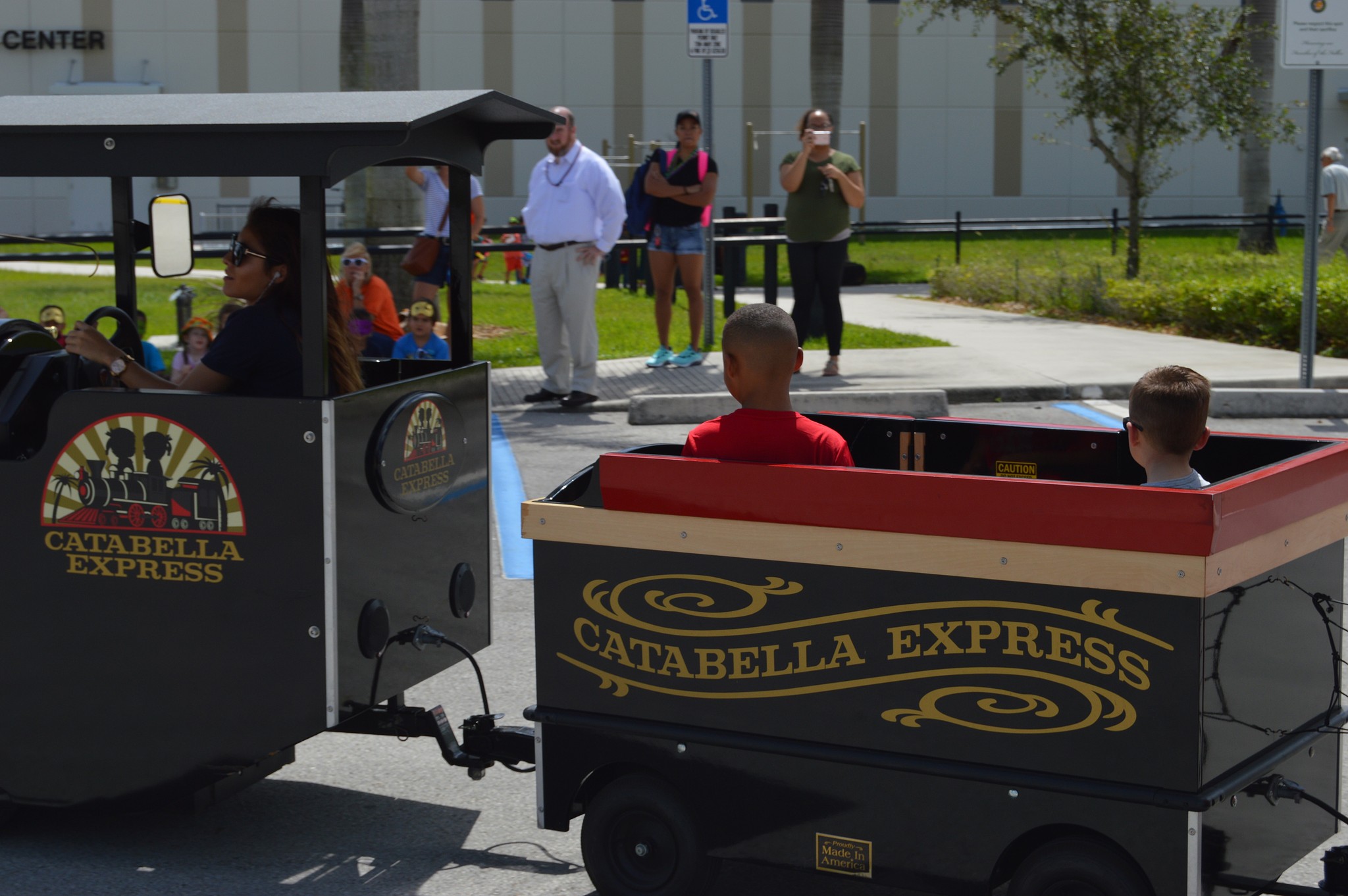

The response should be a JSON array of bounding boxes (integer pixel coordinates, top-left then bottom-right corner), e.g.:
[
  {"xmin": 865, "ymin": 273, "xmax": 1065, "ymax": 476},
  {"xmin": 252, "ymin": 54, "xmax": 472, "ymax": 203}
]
[{"xmin": 544, "ymin": 144, "xmax": 582, "ymax": 186}]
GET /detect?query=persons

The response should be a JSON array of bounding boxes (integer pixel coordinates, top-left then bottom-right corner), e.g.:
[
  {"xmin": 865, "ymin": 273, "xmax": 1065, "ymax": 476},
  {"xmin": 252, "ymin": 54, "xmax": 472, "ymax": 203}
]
[
  {"xmin": 38, "ymin": 196, "xmax": 367, "ymax": 401},
  {"xmin": 1316, "ymin": 146, "xmax": 1348, "ymax": 265},
  {"xmin": 405, "ymin": 159, "xmax": 484, "ymax": 361},
  {"xmin": 1126, "ymin": 365, "xmax": 1212, "ymax": 490},
  {"xmin": 681, "ymin": 303, "xmax": 855, "ymax": 468},
  {"xmin": 521, "ymin": 105, "xmax": 628, "ymax": 410},
  {"xmin": 473, "ymin": 215, "xmax": 533, "ymax": 287},
  {"xmin": 332, "ymin": 241, "xmax": 450, "ymax": 365},
  {"xmin": 645, "ymin": 111, "xmax": 718, "ymax": 370},
  {"xmin": 778, "ymin": 108, "xmax": 865, "ymax": 376}
]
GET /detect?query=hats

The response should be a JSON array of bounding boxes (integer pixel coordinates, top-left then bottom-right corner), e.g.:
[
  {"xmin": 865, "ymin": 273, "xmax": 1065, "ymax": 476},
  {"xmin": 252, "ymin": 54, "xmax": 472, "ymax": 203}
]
[
  {"xmin": 676, "ymin": 111, "xmax": 701, "ymax": 127},
  {"xmin": 40, "ymin": 307, "xmax": 64, "ymax": 324},
  {"xmin": 509, "ymin": 217, "xmax": 519, "ymax": 224},
  {"xmin": 180, "ymin": 316, "xmax": 213, "ymax": 342}
]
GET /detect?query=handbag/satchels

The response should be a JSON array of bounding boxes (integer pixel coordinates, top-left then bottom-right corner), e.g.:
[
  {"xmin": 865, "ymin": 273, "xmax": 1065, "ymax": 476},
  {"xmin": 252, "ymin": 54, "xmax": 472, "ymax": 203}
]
[{"xmin": 400, "ymin": 238, "xmax": 441, "ymax": 275}]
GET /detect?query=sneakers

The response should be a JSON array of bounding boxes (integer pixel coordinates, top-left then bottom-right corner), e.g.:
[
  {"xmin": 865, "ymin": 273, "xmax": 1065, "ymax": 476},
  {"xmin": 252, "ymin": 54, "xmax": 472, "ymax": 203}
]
[
  {"xmin": 669, "ymin": 344, "xmax": 703, "ymax": 367},
  {"xmin": 646, "ymin": 344, "xmax": 674, "ymax": 367}
]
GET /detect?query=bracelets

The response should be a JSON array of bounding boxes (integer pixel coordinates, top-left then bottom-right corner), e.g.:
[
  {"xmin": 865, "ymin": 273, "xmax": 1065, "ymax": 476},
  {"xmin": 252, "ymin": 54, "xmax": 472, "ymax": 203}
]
[{"xmin": 683, "ymin": 185, "xmax": 690, "ymax": 196}]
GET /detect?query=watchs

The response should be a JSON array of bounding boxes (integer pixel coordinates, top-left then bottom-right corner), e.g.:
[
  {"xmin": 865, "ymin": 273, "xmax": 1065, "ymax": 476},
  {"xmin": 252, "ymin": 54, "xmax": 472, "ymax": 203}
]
[
  {"xmin": 351, "ymin": 293, "xmax": 366, "ymax": 303},
  {"xmin": 108, "ymin": 355, "xmax": 136, "ymax": 377}
]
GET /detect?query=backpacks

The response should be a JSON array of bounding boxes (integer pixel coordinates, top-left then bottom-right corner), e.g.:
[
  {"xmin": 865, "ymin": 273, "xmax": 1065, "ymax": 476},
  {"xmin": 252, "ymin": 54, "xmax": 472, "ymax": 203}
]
[{"xmin": 622, "ymin": 148, "xmax": 668, "ymax": 233}]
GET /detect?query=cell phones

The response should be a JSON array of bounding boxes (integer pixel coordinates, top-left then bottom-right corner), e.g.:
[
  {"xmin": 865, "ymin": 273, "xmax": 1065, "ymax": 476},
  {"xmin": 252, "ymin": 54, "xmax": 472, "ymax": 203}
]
[{"xmin": 811, "ymin": 131, "xmax": 832, "ymax": 145}]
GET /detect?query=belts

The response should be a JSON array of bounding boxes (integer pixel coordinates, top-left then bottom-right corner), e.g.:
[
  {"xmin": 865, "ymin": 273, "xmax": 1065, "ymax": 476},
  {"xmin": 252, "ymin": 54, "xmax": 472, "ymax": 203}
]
[{"xmin": 541, "ymin": 240, "xmax": 575, "ymax": 251}]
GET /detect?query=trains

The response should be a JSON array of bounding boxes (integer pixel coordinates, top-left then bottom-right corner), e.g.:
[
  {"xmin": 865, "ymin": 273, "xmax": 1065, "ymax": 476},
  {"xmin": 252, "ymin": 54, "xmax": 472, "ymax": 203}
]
[{"xmin": 0, "ymin": 88, "xmax": 1348, "ymax": 896}]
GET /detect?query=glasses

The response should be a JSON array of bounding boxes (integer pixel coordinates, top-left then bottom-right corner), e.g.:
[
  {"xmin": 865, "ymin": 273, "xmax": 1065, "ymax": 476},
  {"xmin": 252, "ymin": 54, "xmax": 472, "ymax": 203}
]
[
  {"xmin": 1122, "ymin": 417, "xmax": 1143, "ymax": 433},
  {"xmin": 231, "ymin": 235, "xmax": 281, "ymax": 268},
  {"xmin": 341, "ymin": 257, "xmax": 371, "ymax": 268}
]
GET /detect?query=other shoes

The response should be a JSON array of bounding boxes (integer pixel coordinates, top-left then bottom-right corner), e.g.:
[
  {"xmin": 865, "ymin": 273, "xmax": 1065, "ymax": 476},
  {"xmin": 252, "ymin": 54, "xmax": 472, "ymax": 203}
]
[
  {"xmin": 560, "ymin": 390, "xmax": 595, "ymax": 405},
  {"xmin": 825, "ymin": 362, "xmax": 836, "ymax": 377},
  {"xmin": 524, "ymin": 388, "xmax": 563, "ymax": 402}
]
